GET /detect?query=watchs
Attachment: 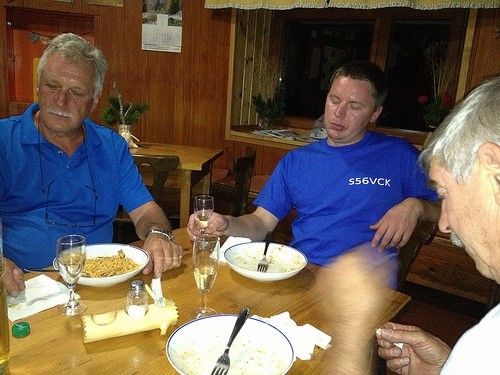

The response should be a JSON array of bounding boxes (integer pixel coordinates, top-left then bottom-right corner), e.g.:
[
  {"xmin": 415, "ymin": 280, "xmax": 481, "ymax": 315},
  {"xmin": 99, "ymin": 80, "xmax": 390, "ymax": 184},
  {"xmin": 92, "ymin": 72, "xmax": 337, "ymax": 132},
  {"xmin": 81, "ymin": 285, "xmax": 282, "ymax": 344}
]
[{"xmin": 145, "ymin": 228, "xmax": 175, "ymax": 242}]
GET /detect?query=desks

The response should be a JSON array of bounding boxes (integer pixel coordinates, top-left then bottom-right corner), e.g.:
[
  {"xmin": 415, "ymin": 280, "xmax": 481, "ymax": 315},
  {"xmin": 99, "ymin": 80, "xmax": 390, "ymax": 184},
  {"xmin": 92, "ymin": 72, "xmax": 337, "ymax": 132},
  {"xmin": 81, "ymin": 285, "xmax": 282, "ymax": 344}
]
[
  {"xmin": 0, "ymin": 226, "xmax": 411, "ymax": 375},
  {"xmin": 125, "ymin": 141, "xmax": 224, "ymax": 228}
]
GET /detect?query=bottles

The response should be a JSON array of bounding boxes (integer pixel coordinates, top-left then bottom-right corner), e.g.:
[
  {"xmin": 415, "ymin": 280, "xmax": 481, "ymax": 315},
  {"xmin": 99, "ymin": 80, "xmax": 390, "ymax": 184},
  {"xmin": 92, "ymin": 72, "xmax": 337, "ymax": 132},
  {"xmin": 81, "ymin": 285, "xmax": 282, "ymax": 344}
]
[{"xmin": 125, "ymin": 279, "xmax": 149, "ymax": 319}]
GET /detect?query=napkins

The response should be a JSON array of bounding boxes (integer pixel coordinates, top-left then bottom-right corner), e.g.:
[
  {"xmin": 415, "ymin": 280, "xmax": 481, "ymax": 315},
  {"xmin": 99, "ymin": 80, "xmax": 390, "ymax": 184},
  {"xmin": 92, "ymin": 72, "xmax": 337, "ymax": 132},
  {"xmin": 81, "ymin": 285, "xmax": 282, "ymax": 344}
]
[
  {"xmin": 210, "ymin": 236, "xmax": 252, "ymax": 264},
  {"xmin": 5, "ymin": 274, "xmax": 81, "ymax": 324},
  {"xmin": 251, "ymin": 309, "xmax": 331, "ymax": 363}
]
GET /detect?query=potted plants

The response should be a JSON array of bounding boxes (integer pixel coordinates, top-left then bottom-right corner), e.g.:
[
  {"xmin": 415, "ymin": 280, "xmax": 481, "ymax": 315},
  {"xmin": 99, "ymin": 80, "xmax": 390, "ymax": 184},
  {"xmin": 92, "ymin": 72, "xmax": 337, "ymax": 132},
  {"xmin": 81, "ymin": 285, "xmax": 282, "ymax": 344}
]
[{"xmin": 100, "ymin": 81, "xmax": 151, "ymax": 148}]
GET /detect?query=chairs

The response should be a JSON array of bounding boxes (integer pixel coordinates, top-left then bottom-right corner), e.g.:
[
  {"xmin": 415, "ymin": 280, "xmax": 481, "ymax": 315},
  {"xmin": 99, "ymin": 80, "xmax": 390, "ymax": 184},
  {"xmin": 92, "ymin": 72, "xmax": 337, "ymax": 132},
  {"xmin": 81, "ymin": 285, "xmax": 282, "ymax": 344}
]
[
  {"xmin": 111, "ymin": 155, "xmax": 179, "ymax": 244},
  {"xmin": 397, "ymin": 222, "xmax": 438, "ymax": 292},
  {"xmin": 230, "ymin": 147, "xmax": 255, "ymax": 217}
]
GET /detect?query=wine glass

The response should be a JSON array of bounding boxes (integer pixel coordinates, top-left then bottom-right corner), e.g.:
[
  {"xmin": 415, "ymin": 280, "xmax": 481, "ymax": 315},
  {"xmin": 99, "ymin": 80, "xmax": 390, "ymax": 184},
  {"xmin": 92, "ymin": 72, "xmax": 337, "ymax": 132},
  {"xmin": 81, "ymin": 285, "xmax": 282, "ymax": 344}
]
[
  {"xmin": 55, "ymin": 234, "xmax": 88, "ymax": 317},
  {"xmin": 193, "ymin": 194, "xmax": 215, "ymax": 250},
  {"xmin": 191, "ymin": 234, "xmax": 221, "ymax": 318}
]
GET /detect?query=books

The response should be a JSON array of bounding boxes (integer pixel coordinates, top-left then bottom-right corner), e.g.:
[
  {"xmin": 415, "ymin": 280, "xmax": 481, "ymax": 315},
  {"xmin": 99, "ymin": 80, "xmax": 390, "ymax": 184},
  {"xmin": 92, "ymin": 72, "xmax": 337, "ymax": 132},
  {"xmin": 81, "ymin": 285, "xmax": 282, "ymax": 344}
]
[{"xmin": 250, "ymin": 129, "xmax": 319, "ymax": 142}]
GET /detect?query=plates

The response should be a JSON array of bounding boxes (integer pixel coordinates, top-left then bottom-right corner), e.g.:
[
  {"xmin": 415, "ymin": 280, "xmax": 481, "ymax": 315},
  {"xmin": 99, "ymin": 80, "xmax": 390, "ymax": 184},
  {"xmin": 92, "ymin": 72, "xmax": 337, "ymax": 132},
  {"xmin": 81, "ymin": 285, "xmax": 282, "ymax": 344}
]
[{"xmin": 165, "ymin": 313, "xmax": 294, "ymax": 375}]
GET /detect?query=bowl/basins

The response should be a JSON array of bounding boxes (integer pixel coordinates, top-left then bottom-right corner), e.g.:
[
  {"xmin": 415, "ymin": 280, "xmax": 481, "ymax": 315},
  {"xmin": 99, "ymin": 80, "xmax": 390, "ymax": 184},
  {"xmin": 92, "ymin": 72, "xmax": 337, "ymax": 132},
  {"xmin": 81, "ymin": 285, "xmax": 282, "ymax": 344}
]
[
  {"xmin": 52, "ymin": 242, "xmax": 150, "ymax": 288},
  {"xmin": 222, "ymin": 241, "xmax": 308, "ymax": 282}
]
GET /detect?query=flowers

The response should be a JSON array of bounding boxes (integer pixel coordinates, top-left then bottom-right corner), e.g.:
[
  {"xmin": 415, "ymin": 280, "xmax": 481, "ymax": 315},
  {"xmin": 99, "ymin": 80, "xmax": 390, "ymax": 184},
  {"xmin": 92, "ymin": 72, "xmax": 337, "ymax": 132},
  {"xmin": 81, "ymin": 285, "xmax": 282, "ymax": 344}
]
[{"xmin": 418, "ymin": 91, "xmax": 453, "ymax": 129}]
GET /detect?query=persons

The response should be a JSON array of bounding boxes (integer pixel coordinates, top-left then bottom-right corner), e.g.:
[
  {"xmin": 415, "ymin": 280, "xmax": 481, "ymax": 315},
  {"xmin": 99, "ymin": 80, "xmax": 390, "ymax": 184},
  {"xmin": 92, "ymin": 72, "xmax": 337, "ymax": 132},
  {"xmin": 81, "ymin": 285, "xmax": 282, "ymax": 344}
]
[
  {"xmin": 0, "ymin": 33, "xmax": 183, "ymax": 298},
  {"xmin": 185, "ymin": 61, "xmax": 442, "ymax": 290},
  {"xmin": 373, "ymin": 78, "xmax": 500, "ymax": 375}
]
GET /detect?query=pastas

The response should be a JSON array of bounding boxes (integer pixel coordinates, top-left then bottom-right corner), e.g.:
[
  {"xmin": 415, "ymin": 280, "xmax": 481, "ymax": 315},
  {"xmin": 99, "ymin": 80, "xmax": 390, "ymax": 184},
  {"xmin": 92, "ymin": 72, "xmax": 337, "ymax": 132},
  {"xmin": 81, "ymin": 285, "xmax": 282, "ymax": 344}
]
[{"xmin": 67, "ymin": 254, "xmax": 138, "ymax": 278}]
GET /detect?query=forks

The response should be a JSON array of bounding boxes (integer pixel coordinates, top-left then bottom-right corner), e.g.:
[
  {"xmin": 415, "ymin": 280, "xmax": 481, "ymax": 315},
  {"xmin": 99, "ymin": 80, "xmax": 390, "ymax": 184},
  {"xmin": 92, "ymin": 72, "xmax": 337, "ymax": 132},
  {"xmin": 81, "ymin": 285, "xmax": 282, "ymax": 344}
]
[
  {"xmin": 256, "ymin": 230, "xmax": 273, "ymax": 273},
  {"xmin": 210, "ymin": 306, "xmax": 250, "ymax": 375}
]
